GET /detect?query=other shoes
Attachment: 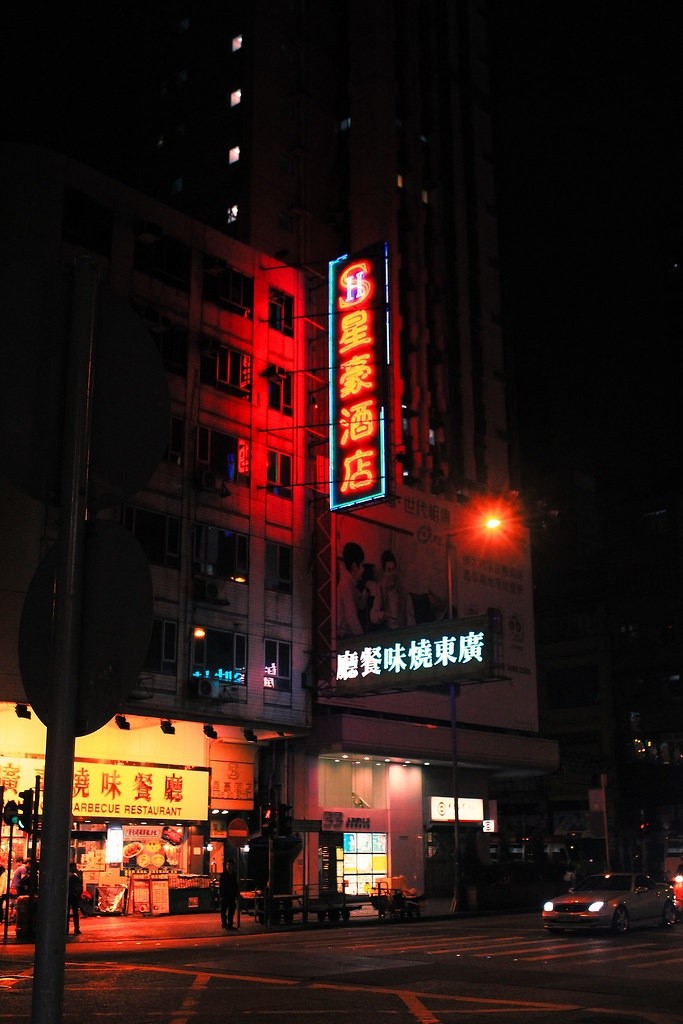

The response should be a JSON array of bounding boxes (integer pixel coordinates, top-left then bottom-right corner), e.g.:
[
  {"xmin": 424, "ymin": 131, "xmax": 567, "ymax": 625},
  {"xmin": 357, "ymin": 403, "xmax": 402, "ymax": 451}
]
[
  {"xmin": 222, "ymin": 926, "xmax": 236, "ymax": 930},
  {"xmin": 74, "ymin": 931, "xmax": 82, "ymax": 935}
]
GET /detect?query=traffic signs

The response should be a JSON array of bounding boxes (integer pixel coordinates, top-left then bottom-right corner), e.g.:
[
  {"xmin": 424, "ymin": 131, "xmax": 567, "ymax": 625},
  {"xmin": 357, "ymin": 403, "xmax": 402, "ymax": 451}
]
[{"xmin": 226, "ymin": 818, "xmax": 250, "ymax": 848}]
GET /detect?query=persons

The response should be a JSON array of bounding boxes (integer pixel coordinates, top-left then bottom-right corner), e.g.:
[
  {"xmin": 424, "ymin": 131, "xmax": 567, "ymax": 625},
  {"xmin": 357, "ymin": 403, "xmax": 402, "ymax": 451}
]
[
  {"xmin": 15, "ymin": 857, "xmax": 25, "ymax": 867},
  {"xmin": 0, "ymin": 866, "xmax": 8, "ymax": 924},
  {"xmin": 65, "ymin": 863, "xmax": 83, "ymax": 933},
  {"xmin": 10, "ymin": 857, "xmax": 32, "ymax": 897},
  {"xmin": 218, "ymin": 860, "xmax": 241, "ymax": 930}
]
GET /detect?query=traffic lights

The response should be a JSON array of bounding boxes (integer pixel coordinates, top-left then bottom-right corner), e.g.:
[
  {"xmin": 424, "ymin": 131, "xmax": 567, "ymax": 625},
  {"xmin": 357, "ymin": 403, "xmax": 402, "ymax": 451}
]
[
  {"xmin": 17, "ymin": 790, "xmax": 33, "ymax": 834},
  {"xmin": 261, "ymin": 801, "xmax": 275, "ymax": 839}
]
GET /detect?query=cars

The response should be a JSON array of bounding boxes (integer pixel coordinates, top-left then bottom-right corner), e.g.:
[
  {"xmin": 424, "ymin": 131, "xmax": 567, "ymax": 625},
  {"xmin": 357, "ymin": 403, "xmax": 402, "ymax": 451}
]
[{"xmin": 541, "ymin": 873, "xmax": 677, "ymax": 935}]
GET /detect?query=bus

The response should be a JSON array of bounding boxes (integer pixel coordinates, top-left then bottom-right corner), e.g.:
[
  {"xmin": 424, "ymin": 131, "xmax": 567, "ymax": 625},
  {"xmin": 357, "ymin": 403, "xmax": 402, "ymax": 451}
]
[{"xmin": 663, "ymin": 827, "xmax": 683, "ymax": 884}]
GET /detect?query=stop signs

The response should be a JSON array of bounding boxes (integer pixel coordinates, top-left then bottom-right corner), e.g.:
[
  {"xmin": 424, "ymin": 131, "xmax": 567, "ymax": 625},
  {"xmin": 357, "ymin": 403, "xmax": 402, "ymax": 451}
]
[{"xmin": 4, "ymin": 801, "xmax": 18, "ymax": 825}]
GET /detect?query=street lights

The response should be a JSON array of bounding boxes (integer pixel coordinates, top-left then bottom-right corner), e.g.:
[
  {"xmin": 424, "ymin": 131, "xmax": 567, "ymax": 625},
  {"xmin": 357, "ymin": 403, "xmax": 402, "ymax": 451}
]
[{"xmin": 446, "ymin": 516, "xmax": 504, "ymax": 917}]
